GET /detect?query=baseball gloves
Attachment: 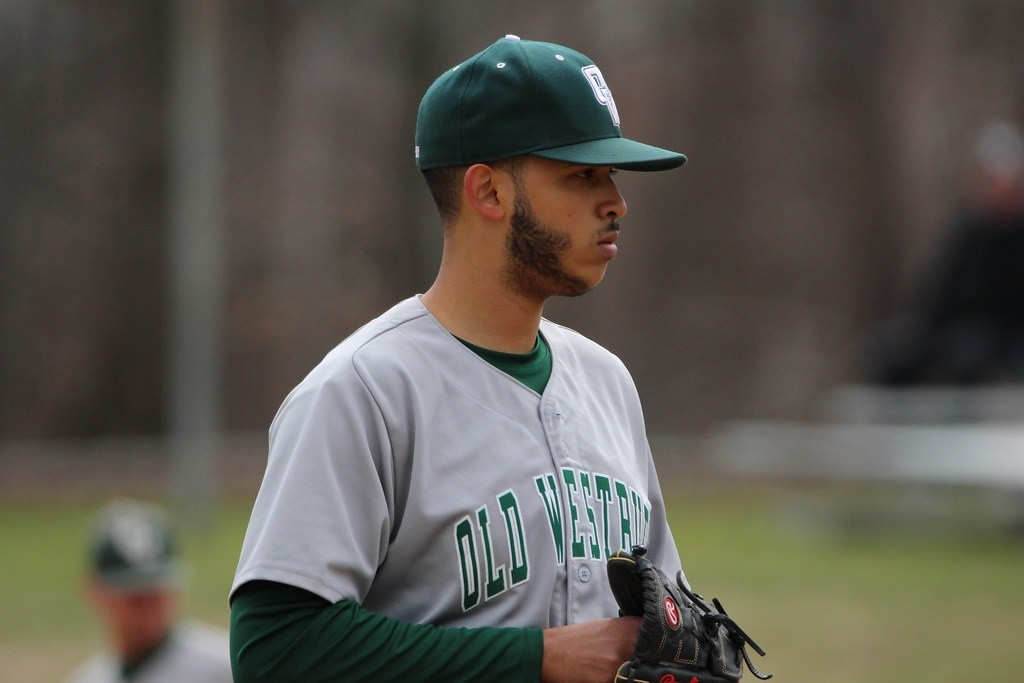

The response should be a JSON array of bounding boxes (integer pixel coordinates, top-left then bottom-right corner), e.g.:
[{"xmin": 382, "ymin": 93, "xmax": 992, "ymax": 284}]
[{"xmin": 606, "ymin": 544, "xmax": 746, "ymax": 683}]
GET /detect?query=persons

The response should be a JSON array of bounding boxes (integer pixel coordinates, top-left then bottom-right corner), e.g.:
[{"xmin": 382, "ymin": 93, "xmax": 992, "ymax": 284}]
[
  {"xmin": 227, "ymin": 33, "xmax": 774, "ymax": 683},
  {"xmin": 68, "ymin": 498, "xmax": 232, "ymax": 683}
]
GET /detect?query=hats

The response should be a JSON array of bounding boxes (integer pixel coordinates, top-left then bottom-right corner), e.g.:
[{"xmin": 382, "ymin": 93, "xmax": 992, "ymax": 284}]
[
  {"xmin": 86, "ymin": 504, "xmax": 186, "ymax": 590},
  {"xmin": 414, "ymin": 35, "xmax": 688, "ymax": 172}
]
